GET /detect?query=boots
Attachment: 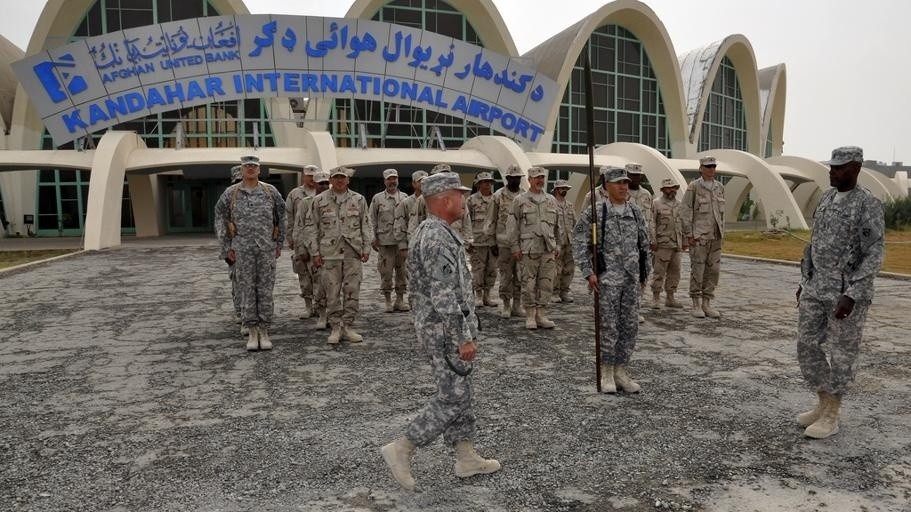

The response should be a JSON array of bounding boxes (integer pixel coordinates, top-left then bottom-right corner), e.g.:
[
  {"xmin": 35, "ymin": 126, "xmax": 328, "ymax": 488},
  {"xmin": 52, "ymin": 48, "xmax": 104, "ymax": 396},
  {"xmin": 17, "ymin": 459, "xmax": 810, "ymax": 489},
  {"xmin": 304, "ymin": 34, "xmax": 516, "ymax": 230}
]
[
  {"xmin": 380, "ymin": 435, "xmax": 418, "ymax": 490},
  {"xmin": 652, "ymin": 289, "xmax": 662, "ymax": 310},
  {"xmin": 690, "ymin": 295, "xmax": 706, "ymax": 319},
  {"xmin": 666, "ymin": 290, "xmax": 683, "ymax": 308},
  {"xmin": 450, "ymin": 440, "xmax": 501, "ymax": 479},
  {"xmin": 795, "ymin": 389, "xmax": 844, "ymax": 441},
  {"xmin": 236, "ymin": 313, "xmax": 273, "ymax": 352},
  {"xmin": 613, "ymin": 362, "xmax": 641, "ymax": 394},
  {"xmin": 474, "ymin": 286, "xmax": 575, "ymax": 331},
  {"xmin": 300, "ymin": 293, "xmax": 363, "ymax": 345},
  {"xmin": 599, "ymin": 361, "xmax": 617, "ymax": 395},
  {"xmin": 383, "ymin": 292, "xmax": 411, "ymax": 313},
  {"xmin": 701, "ymin": 295, "xmax": 721, "ymax": 318}
]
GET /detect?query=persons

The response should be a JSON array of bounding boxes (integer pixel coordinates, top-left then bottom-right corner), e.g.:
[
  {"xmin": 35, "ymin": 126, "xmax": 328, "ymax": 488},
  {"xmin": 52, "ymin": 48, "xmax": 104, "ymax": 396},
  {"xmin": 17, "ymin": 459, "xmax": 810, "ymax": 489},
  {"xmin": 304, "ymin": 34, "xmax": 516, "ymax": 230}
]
[
  {"xmin": 380, "ymin": 173, "xmax": 500, "ymax": 485},
  {"xmin": 215, "ymin": 155, "xmax": 474, "ymax": 351},
  {"xmin": 465, "ymin": 157, "xmax": 725, "ymax": 330},
  {"xmin": 796, "ymin": 146, "xmax": 885, "ymax": 439},
  {"xmin": 572, "ymin": 169, "xmax": 653, "ymax": 393}
]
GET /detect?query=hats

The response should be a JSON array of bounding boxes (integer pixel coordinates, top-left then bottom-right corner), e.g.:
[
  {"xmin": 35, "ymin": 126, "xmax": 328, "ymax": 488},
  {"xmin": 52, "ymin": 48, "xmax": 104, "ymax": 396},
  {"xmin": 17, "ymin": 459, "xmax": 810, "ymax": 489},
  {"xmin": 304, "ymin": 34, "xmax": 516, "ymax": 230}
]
[
  {"xmin": 698, "ymin": 156, "xmax": 717, "ymax": 167},
  {"xmin": 380, "ymin": 165, "xmax": 572, "ymax": 197},
  {"xmin": 598, "ymin": 162, "xmax": 644, "ymax": 188},
  {"xmin": 659, "ymin": 177, "xmax": 681, "ymax": 191},
  {"xmin": 230, "ymin": 166, "xmax": 243, "ymax": 182},
  {"xmin": 240, "ymin": 156, "xmax": 260, "ymax": 167},
  {"xmin": 818, "ymin": 145, "xmax": 864, "ymax": 167},
  {"xmin": 303, "ymin": 164, "xmax": 350, "ymax": 183}
]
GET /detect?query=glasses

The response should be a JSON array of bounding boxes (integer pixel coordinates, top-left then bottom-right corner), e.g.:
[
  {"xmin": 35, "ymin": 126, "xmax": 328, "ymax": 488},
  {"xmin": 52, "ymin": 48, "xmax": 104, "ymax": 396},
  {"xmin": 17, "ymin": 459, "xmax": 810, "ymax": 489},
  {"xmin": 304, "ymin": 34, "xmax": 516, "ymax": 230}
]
[{"xmin": 702, "ymin": 163, "xmax": 717, "ymax": 169}]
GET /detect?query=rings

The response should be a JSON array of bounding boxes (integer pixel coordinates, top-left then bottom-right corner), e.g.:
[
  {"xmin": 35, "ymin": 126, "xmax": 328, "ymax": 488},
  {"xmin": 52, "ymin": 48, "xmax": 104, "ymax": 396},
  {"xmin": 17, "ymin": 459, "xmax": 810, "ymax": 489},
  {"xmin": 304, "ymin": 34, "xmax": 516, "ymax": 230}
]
[{"xmin": 844, "ymin": 314, "xmax": 847, "ymax": 317}]
